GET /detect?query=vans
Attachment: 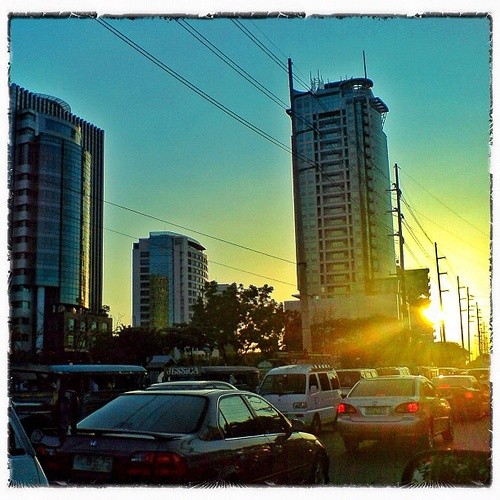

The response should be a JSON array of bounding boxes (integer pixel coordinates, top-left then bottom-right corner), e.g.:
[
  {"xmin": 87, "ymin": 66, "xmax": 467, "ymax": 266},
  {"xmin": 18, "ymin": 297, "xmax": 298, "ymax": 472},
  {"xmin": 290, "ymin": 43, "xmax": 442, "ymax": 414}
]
[{"xmin": 256, "ymin": 363, "xmax": 343, "ymax": 437}]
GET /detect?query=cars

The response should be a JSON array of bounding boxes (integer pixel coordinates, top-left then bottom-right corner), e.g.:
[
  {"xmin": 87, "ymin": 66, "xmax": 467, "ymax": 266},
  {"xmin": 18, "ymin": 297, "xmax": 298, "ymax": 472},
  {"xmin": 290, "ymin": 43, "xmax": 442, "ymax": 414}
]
[
  {"xmin": 30, "ymin": 387, "xmax": 329, "ymax": 486},
  {"xmin": 336, "ymin": 375, "xmax": 454, "ymax": 454},
  {"xmin": 429, "ymin": 374, "xmax": 482, "ymax": 420}
]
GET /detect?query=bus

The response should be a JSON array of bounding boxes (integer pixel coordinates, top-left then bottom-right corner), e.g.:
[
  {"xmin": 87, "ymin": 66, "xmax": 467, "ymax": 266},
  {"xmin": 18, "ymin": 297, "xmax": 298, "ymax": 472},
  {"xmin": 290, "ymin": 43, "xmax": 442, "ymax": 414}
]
[{"xmin": 11, "ymin": 363, "xmax": 148, "ymax": 425}]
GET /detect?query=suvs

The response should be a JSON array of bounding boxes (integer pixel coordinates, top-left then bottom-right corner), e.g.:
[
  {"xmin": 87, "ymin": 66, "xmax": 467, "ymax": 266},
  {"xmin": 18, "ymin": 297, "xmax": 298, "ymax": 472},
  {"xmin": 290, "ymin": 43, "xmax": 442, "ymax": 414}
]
[{"xmin": 335, "ymin": 368, "xmax": 379, "ymax": 400}]
